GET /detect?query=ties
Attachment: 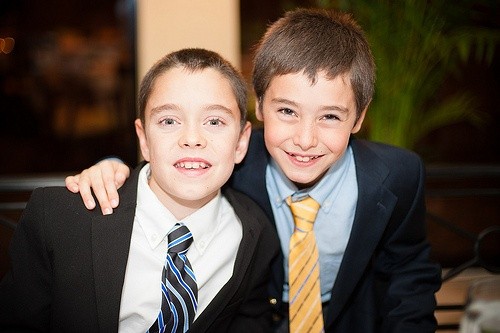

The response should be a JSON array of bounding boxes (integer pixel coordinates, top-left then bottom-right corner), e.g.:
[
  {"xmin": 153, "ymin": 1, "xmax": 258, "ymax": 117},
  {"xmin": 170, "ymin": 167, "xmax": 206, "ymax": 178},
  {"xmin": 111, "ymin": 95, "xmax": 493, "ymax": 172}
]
[
  {"xmin": 146, "ymin": 224, "xmax": 198, "ymax": 333},
  {"xmin": 285, "ymin": 196, "xmax": 325, "ymax": 333}
]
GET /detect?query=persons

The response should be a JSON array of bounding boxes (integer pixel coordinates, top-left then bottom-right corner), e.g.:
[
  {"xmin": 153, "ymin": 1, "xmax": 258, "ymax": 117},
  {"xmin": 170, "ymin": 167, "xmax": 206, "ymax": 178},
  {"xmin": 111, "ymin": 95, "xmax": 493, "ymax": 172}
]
[
  {"xmin": 63, "ymin": 8, "xmax": 440, "ymax": 332},
  {"xmin": 0, "ymin": 48, "xmax": 284, "ymax": 333}
]
